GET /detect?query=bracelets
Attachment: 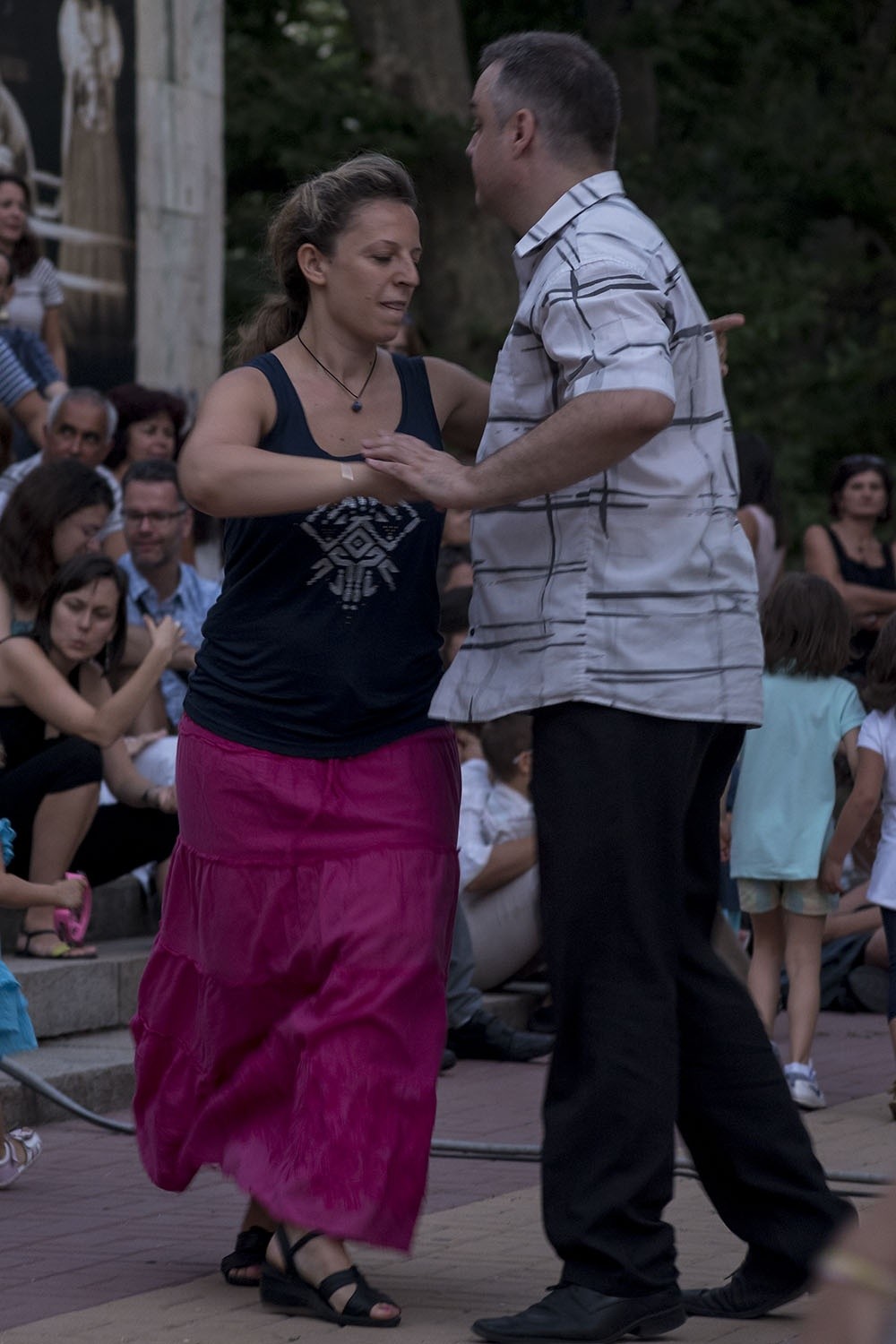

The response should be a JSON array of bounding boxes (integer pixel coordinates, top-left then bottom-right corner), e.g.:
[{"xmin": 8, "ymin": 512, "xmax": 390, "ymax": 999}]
[{"xmin": 339, "ymin": 460, "xmax": 356, "ymax": 502}]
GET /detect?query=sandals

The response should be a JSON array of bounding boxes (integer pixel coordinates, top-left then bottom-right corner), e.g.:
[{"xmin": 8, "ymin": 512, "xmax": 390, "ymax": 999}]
[
  {"xmin": 15, "ymin": 922, "xmax": 97, "ymax": 959},
  {"xmin": 220, "ymin": 1225, "xmax": 273, "ymax": 1285},
  {"xmin": 262, "ymin": 1221, "xmax": 402, "ymax": 1327}
]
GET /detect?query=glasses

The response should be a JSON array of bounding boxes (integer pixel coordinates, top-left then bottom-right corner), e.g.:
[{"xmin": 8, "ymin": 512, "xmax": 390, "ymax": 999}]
[
  {"xmin": 845, "ymin": 456, "xmax": 883, "ymax": 465},
  {"xmin": 121, "ymin": 508, "xmax": 189, "ymax": 527}
]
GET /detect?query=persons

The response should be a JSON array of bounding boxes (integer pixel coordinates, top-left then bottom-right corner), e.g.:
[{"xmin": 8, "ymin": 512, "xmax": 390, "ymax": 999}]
[
  {"xmin": 0, "ymin": 547, "xmax": 188, "ymax": 959},
  {"xmin": 0, "ymin": 182, "xmax": 895, "ymax": 1113},
  {"xmin": 365, "ymin": 34, "xmax": 859, "ymax": 1344},
  {"xmin": 124, "ymin": 154, "xmax": 494, "ymax": 1327}
]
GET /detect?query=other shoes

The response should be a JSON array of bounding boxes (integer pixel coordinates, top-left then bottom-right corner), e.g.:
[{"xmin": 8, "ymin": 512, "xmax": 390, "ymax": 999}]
[
  {"xmin": 439, "ymin": 1050, "xmax": 456, "ymax": 1071},
  {"xmin": 447, "ymin": 1009, "xmax": 555, "ymax": 1061},
  {"xmin": 0, "ymin": 1126, "xmax": 42, "ymax": 1187}
]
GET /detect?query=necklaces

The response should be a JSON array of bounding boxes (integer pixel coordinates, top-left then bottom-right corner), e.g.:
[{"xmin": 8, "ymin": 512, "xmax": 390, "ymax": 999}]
[{"xmin": 296, "ymin": 330, "xmax": 379, "ymax": 412}]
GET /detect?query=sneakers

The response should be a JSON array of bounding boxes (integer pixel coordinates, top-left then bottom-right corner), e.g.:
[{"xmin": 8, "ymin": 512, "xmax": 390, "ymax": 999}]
[{"xmin": 781, "ymin": 1057, "xmax": 826, "ymax": 1108}]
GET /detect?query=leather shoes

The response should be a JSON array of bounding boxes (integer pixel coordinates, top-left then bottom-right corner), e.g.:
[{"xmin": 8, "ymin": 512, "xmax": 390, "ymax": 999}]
[
  {"xmin": 469, "ymin": 1283, "xmax": 687, "ymax": 1344},
  {"xmin": 682, "ymin": 1203, "xmax": 862, "ymax": 1317}
]
